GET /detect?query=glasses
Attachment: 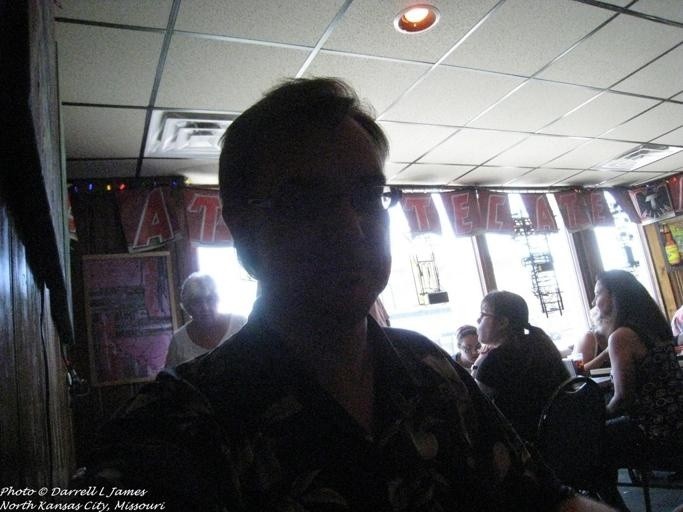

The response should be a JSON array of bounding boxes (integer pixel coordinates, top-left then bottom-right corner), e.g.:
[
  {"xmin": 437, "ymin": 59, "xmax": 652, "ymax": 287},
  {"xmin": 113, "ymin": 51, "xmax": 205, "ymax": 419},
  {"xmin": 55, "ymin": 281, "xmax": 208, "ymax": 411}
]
[
  {"xmin": 480, "ymin": 311, "xmax": 494, "ymax": 319},
  {"xmin": 234, "ymin": 185, "xmax": 401, "ymax": 218},
  {"xmin": 459, "ymin": 343, "xmax": 481, "ymax": 353}
]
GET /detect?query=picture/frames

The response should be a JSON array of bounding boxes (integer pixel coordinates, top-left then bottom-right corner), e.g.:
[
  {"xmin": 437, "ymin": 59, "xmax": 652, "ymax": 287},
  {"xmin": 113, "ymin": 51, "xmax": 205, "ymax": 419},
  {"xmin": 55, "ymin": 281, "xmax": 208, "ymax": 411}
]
[{"xmin": 82, "ymin": 250, "xmax": 177, "ymax": 387}]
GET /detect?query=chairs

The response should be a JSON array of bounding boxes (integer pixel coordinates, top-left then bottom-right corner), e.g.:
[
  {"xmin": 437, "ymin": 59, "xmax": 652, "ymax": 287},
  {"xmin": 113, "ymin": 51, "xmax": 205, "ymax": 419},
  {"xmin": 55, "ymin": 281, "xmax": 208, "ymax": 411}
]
[
  {"xmin": 533, "ymin": 376, "xmax": 607, "ymax": 502},
  {"xmin": 618, "ymin": 356, "xmax": 683, "ymax": 512}
]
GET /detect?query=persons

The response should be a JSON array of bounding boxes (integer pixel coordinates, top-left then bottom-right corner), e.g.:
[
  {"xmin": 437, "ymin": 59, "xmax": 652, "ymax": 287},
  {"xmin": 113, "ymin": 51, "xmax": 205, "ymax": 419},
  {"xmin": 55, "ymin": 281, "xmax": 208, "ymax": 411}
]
[
  {"xmin": 90, "ymin": 78, "xmax": 683, "ymax": 512},
  {"xmin": 591, "ymin": 270, "xmax": 683, "ymax": 512},
  {"xmin": 470, "ymin": 290, "xmax": 574, "ymax": 448},
  {"xmin": 571, "ymin": 307, "xmax": 611, "ymax": 370},
  {"xmin": 450, "ymin": 325, "xmax": 482, "ymax": 376},
  {"xmin": 165, "ymin": 271, "xmax": 248, "ymax": 372}
]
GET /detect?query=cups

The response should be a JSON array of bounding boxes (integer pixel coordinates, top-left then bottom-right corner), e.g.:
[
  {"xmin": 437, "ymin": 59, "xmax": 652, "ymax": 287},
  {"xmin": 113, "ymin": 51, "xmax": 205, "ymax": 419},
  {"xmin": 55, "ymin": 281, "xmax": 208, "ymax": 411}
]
[{"xmin": 567, "ymin": 353, "xmax": 584, "ymax": 375}]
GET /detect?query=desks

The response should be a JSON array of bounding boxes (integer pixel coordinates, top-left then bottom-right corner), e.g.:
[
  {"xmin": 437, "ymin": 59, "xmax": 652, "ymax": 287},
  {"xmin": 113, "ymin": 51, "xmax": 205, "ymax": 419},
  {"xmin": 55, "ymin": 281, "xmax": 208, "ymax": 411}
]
[{"xmin": 588, "ymin": 346, "xmax": 683, "ymax": 389}]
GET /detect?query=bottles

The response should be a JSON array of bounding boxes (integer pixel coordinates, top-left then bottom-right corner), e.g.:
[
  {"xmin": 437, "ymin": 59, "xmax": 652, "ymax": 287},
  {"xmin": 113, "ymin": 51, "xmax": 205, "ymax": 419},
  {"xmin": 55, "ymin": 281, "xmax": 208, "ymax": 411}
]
[{"xmin": 661, "ymin": 222, "xmax": 682, "ymax": 267}]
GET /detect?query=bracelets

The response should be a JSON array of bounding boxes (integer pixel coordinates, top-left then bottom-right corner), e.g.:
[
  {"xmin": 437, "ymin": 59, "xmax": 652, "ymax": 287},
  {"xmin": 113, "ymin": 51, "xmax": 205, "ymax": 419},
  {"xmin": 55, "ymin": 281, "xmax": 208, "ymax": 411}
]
[{"xmin": 470, "ymin": 365, "xmax": 479, "ymax": 370}]
